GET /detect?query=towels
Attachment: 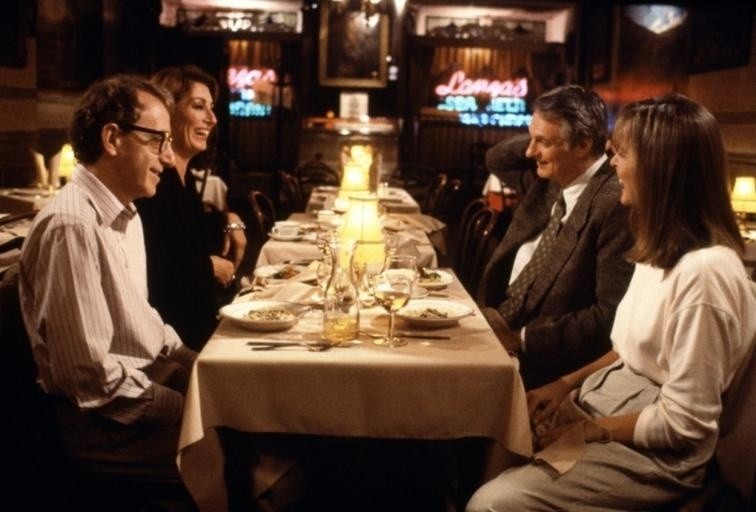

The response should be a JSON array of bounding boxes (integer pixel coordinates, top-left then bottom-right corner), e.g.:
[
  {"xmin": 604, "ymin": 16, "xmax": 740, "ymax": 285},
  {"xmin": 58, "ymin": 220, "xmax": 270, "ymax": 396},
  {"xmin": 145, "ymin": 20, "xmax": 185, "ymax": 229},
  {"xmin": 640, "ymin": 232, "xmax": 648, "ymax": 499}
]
[
  {"xmin": 251, "ymin": 281, "xmax": 324, "ymax": 307},
  {"xmin": 288, "ymin": 259, "xmax": 325, "ymax": 282}
]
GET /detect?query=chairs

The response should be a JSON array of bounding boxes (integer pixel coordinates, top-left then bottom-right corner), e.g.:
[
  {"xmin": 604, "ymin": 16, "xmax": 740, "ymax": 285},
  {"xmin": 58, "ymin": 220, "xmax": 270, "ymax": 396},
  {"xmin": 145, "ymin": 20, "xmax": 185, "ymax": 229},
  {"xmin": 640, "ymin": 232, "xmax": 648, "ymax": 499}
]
[
  {"xmin": 1, "ymin": 266, "xmax": 194, "ymax": 511},
  {"xmin": 276, "ymin": 167, "xmax": 307, "ymax": 213},
  {"xmin": 248, "ymin": 189, "xmax": 278, "ymax": 259},
  {"xmin": 667, "ymin": 348, "xmax": 756, "ymax": 511},
  {"xmin": 405, "ymin": 159, "xmax": 501, "ymax": 289}
]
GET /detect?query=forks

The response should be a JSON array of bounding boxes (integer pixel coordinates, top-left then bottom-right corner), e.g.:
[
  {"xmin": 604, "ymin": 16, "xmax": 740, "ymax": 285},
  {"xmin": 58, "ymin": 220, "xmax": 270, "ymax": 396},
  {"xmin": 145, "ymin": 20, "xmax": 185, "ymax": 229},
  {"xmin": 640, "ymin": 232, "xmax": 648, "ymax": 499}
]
[
  {"xmin": 392, "ymin": 333, "xmax": 451, "ymax": 341},
  {"xmin": 251, "ymin": 340, "xmax": 340, "ymax": 351}
]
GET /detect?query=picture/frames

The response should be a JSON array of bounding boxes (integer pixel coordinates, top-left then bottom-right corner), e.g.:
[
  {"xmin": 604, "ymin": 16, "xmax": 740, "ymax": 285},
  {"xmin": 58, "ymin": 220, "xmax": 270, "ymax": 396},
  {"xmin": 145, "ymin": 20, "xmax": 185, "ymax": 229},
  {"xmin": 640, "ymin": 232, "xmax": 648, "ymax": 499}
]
[{"xmin": 316, "ymin": 0, "xmax": 392, "ymax": 88}]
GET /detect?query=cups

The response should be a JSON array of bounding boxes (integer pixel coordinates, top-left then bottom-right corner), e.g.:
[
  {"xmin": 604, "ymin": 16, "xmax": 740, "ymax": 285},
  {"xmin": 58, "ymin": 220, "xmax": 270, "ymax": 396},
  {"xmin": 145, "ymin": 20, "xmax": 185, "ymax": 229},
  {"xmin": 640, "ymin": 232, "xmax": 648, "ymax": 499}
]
[{"xmin": 272, "ymin": 222, "xmax": 299, "ymax": 235}]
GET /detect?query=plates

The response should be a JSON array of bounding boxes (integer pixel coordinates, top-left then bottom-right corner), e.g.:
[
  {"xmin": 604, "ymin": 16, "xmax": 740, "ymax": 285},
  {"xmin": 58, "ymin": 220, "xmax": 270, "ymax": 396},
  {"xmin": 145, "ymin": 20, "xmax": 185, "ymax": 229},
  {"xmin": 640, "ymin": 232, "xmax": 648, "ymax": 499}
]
[
  {"xmin": 395, "ymin": 299, "xmax": 474, "ymax": 328},
  {"xmin": 218, "ymin": 300, "xmax": 311, "ymax": 331},
  {"xmin": 254, "ymin": 264, "xmax": 307, "ymax": 286},
  {"xmin": 267, "ymin": 231, "xmax": 304, "ymax": 241},
  {"xmin": 415, "ymin": 287, "xmax": 429, "ymax": 298},
  {"xmin": 415, "ymin": 269, "xmax": 453, "ymax": 290}
]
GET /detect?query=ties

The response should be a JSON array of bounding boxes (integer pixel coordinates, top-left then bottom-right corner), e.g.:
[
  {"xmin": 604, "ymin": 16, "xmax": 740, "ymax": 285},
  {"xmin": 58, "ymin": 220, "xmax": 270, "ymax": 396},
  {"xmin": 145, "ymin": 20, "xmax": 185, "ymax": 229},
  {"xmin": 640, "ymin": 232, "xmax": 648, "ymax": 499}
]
[{"xmin": 499, "ymin": 193, "xmax": 566, "ymax": 325}]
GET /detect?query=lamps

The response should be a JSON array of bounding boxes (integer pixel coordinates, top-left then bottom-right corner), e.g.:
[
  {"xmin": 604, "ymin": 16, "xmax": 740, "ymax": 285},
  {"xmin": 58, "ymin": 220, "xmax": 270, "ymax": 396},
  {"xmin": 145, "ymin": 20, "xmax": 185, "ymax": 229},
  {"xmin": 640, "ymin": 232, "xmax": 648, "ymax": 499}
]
[
  {"xmin": 56, "ymin": 142, "xmax": 75, "ymax": 188},
  {"xmin": 337, "ymin": 192, "xmax": 386, "ymax": 274},
  {"xmin": 328, "ymin": 138, "xmax": 378, "ymax": 213},
  {"xmin": 727, "ymin": 176, "xmax": 756, "ymax": 215}
]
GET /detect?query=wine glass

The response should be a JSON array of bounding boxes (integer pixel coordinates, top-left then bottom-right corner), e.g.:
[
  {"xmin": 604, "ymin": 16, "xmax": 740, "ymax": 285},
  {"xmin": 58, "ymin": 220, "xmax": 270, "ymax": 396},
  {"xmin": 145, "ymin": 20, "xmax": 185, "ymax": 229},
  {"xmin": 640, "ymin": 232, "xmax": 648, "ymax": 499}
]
[
  {"xmin": 374, "ymin": 277, "xmax": 412, "ymax": 348},
  {"xmin": 384, "ymin": 255, "xmax": 417, "ymax": 292}
]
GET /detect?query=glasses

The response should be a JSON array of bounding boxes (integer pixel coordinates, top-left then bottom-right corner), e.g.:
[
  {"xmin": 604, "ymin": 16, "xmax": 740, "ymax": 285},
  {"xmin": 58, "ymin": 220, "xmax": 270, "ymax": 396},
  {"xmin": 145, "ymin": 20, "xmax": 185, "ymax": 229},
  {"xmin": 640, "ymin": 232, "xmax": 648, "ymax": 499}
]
[{"xmin": 123, "ymin": 124, "xmax": 174, "ymax": 153}]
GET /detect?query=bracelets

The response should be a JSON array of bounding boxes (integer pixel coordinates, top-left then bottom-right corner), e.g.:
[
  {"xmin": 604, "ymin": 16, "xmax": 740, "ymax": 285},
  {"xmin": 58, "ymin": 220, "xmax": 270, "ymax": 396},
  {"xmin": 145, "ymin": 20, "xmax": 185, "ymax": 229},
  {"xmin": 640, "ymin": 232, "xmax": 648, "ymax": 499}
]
[{"xmin": 222, "ymin": 221, "xmax": 247, "ymax": 234}]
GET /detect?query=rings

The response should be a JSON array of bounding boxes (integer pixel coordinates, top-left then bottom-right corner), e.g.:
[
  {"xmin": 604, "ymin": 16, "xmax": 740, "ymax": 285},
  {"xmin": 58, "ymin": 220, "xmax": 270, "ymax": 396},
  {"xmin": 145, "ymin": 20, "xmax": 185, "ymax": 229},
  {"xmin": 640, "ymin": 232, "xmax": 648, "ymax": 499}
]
[{"xmin": 231, "ymin": 274, "xmax": 236, "ymax": 280}]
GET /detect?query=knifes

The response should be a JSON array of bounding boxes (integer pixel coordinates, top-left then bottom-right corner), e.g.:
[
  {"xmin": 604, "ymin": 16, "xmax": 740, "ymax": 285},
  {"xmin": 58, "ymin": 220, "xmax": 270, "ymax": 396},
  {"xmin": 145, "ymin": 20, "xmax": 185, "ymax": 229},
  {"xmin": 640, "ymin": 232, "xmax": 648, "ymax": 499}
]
[{"xmin": 247, "ymin": 342, "xmax": 328, "ymax": 346}]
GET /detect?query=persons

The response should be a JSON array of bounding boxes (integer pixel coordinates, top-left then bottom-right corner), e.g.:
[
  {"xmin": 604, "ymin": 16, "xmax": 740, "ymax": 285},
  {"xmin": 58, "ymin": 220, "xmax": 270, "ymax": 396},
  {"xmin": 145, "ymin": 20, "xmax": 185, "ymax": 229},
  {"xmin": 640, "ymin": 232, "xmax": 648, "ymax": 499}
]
[
  {"xmin": 464, "ymin": 91, "xmax": 756, "ymax": 511},
  {"xmin": 132, "ymin": 64, "xmax": 248, "ymax": 337},
  {"xmin": 483, "ymin": 84, "xmax": 640, "ymax": 388},
  {"xmin": 16, "ymin": 75, "xmax": 297, "ymax": 512}
]
